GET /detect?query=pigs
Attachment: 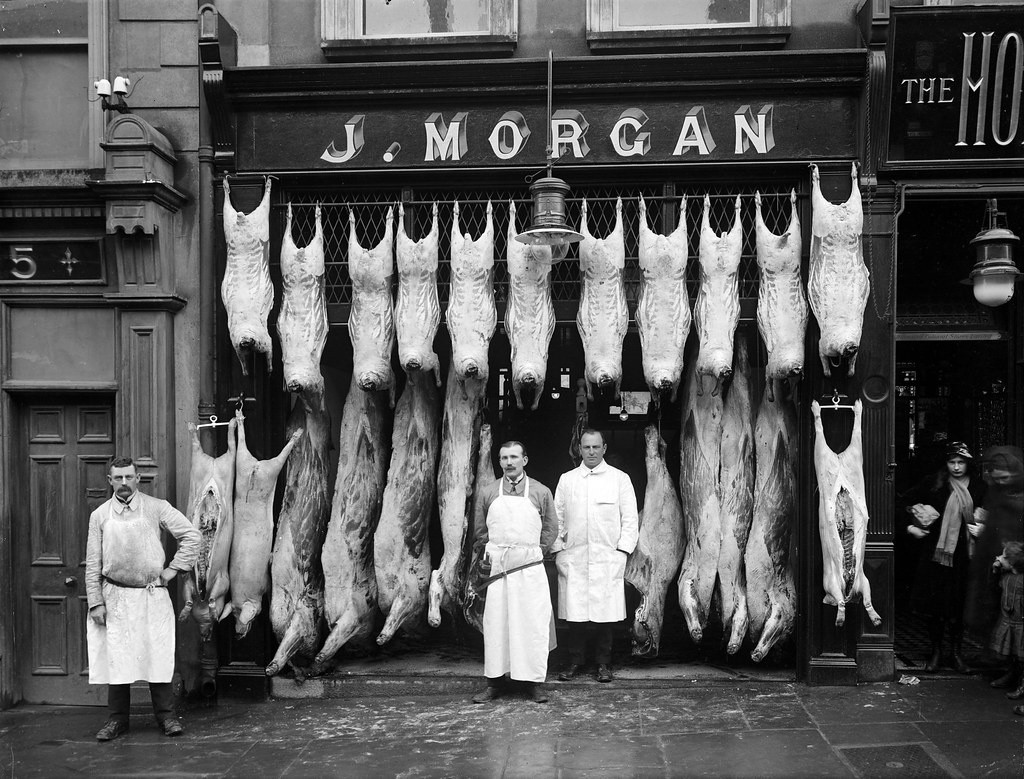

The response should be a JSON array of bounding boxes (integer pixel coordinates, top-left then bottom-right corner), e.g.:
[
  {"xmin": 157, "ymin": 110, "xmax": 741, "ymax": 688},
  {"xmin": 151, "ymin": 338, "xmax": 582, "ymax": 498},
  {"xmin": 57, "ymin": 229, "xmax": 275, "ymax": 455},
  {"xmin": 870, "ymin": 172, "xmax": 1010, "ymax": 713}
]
[
  {"xmin": 177, "ymin": 408, "xmax": 305, "ymax": 637},
  {"xmin": 811, "ymin": 399, "xmax": 883, "ymax": 629}
]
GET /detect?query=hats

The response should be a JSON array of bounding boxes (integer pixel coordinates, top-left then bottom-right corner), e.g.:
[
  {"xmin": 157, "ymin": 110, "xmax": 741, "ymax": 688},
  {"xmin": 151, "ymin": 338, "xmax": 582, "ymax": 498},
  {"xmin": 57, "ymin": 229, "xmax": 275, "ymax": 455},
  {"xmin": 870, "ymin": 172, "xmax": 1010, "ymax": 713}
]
[{"xmin": 943, "ymin": 442, "xmax": 973, "ymax": 458}]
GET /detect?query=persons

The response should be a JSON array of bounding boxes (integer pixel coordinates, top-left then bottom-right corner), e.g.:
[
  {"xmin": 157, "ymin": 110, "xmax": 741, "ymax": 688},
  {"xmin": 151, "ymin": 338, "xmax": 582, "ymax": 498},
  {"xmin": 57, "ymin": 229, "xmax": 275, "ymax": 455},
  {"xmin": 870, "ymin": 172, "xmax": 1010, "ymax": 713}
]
[
  {"xmin": 473, "ymin": 440, "xmax": 560, "ymax": 702},
  {"xmin": 553, "ymin": 427, "xmax": 640, "ymax": 681},
  {"xmin": 906, "ymin": 444, "xmax": 988, "ymax": 676},
  {"xmin": 976, "ymin": 446, "xmax": 1024, "ymax": 716},
  {"xmin": 85, "ymin": 457, "xmax": 201, "ymax": 741}
]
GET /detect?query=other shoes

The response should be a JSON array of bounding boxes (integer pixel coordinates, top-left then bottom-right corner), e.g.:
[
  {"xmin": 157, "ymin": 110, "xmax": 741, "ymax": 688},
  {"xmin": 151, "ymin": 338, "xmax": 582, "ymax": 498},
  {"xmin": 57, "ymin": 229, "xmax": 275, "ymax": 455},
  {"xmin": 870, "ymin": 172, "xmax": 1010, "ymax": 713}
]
[{"xmin": 1013, "ymin": 704, "xmax": 1024, "ymax": 716}]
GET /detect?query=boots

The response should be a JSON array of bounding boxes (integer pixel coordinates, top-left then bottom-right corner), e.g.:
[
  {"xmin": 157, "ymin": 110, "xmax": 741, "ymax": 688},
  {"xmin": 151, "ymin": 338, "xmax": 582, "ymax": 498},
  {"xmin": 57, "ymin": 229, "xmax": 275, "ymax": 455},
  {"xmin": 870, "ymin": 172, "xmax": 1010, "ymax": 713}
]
[
  {"xmin": 949, "ymin": 640, "xmax": 976, "ymax": 675},
  {"xmin": 926, "ymin": 640, "xmax": 942, "ymax": 674},
  {"xmin": 990, "ymin": 662, "xmax": 1024, "ymax": 699}
]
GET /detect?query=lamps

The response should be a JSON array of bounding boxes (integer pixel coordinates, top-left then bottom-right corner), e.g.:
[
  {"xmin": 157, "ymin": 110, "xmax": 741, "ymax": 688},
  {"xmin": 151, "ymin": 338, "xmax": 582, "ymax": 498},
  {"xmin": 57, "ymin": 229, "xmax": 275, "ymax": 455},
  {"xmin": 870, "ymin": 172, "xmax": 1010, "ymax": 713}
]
[
  {"xmin": 970, "ymin": 197, "xmax": 1022, "ymax": 305},
  {"xmin": 93, "ymin": 76, "xmax": 132, "ymax": 113},
  {"xmin": 512, "ymin": 50, "xmax": 585, "ymax": 265}
]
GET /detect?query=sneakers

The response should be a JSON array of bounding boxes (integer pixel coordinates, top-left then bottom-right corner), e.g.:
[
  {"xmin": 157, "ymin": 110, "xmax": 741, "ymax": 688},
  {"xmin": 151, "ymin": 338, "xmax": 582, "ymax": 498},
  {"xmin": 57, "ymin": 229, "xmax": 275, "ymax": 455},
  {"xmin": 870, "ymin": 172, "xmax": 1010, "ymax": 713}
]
[
  {"xmin": 593, "ymin": 664, "xmax": 612, "ymax": 682},
  {"xmin": 472, "ymin": 686, "xmax": 505, "ymax": 702},
  {"xmin": 559, "ymin": 664, "xmax": 579, "ymax": 680},
  {"xmin": 96, "ymin": 719, "xmax": 127, "ymax": 739},
  {"xmin": 528, "ymin": 684, "xmax": 548, "ymax": 703},
  {"xmin": 159, "ymin": 718, "xmax": 181, "ymax": 735}
]
